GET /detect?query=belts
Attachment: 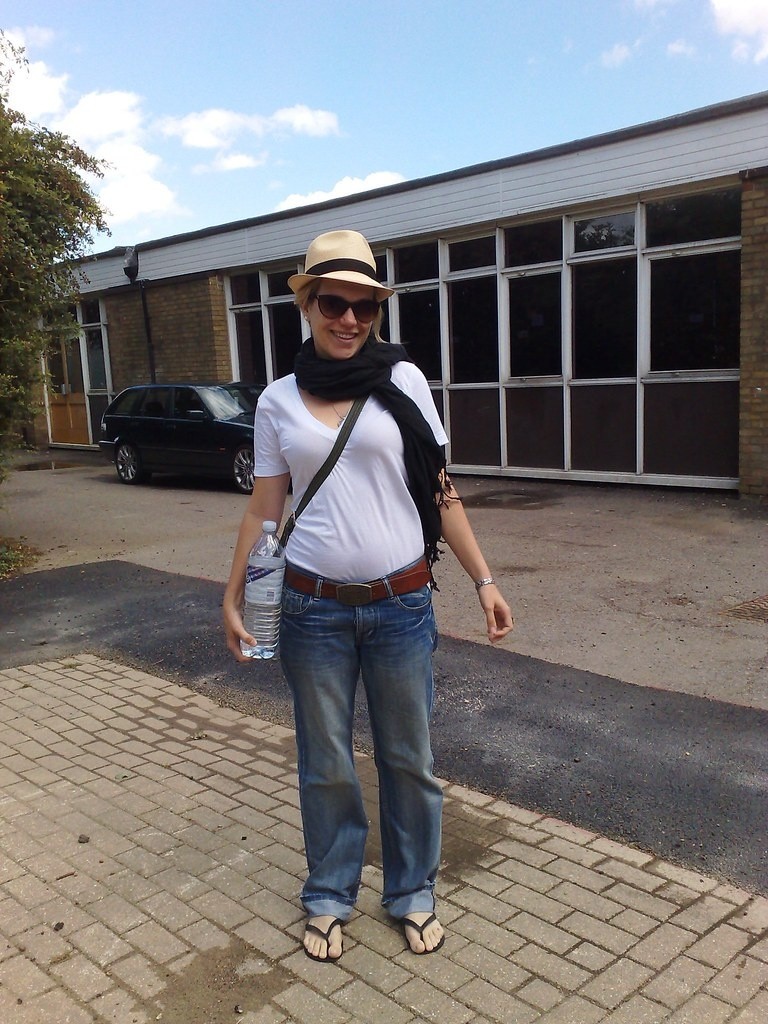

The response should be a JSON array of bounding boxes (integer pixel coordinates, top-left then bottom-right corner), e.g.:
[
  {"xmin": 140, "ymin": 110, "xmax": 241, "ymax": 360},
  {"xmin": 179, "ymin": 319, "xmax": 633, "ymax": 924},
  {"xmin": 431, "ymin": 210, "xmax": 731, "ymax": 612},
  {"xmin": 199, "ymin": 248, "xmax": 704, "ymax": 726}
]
[{"xmin": 284, "ymin": 559, "xmax": 432, "ymax": 607}]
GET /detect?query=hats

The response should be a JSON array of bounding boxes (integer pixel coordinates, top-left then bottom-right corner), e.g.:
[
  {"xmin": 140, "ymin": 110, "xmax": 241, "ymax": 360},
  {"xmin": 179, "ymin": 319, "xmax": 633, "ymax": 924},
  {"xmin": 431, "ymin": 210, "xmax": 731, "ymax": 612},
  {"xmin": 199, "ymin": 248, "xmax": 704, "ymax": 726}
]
[{"xmin": 287, "ymin": 230, "xmax": 394, "ymax": 303}]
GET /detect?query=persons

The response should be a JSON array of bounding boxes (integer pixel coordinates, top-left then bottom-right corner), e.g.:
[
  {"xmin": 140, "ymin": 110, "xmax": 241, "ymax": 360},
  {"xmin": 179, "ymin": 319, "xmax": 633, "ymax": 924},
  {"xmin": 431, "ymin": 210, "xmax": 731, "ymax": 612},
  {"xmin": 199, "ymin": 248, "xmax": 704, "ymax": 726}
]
[{"xmin": 221, "ymin": 230, "xmax": 517, "ymax": 961}]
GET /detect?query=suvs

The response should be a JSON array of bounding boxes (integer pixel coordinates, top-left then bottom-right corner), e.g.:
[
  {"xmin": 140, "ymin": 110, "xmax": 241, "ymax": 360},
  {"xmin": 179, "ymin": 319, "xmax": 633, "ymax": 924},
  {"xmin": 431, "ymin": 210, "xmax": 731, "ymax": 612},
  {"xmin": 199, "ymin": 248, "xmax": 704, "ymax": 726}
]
[{"xmin": 98, "ymin": 379, "xmax": 296, "ymax": 495}]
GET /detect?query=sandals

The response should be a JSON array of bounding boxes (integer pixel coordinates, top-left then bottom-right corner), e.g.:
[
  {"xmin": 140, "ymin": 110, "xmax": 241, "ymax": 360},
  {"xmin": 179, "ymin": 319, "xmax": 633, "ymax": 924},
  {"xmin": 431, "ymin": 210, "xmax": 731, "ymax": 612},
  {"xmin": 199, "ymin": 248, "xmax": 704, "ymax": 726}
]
[
  {"xmin": 402, "ymin": 912, "xmax": 445, "ymax": 955},
  {"xmin": 303, "ymin": 915, "xmax": 343, "ymax": 963}
]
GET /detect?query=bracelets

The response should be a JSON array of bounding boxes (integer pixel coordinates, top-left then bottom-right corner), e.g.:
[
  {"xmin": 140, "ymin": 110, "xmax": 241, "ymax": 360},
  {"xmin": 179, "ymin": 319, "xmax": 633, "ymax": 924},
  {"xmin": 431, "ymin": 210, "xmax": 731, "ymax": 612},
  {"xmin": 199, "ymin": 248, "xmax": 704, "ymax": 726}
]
[{"xmin": 474, "ymin": 577, "xmax": 496, "ymax": 591}]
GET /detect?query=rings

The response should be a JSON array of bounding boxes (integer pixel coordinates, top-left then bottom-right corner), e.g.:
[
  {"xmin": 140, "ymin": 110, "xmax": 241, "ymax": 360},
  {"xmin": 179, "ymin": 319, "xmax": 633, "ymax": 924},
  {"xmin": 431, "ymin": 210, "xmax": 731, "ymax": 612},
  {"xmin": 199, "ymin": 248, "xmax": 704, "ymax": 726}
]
[{"xmin": 511, "ymin": 617, "xmax": 514, "ymax": 624}]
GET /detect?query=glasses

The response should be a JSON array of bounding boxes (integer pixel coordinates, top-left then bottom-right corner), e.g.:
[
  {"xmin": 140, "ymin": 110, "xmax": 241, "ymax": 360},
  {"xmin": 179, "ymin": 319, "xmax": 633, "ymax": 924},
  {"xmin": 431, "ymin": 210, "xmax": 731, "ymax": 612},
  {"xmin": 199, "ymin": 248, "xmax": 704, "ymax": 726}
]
[{"xmin": 315, "ymin": 294, "xmax": 380, "ymax": 323}]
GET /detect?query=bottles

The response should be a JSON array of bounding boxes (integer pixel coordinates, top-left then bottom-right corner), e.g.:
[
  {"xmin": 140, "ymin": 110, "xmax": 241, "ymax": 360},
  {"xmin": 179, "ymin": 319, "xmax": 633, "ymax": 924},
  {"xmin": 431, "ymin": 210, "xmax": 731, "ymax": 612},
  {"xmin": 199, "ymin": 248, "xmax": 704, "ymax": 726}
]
[{"xmin": 239, "ymin": 520, "xmax": 285, "ymax": 660}]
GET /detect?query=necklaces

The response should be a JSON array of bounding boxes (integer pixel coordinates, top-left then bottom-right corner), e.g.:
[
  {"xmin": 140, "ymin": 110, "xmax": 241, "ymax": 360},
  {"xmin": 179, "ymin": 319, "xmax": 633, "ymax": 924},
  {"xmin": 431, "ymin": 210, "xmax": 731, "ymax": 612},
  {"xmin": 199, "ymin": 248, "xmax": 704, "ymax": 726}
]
[{"xmin": 334, "ymin": 400, "xmax": 354, "ymax": 427}]
